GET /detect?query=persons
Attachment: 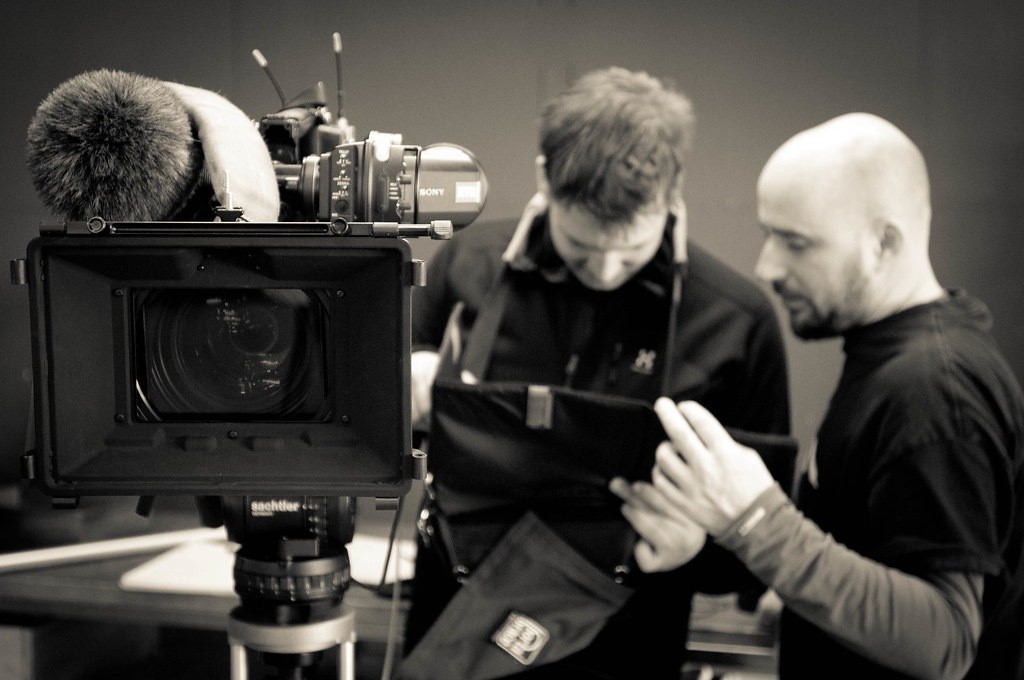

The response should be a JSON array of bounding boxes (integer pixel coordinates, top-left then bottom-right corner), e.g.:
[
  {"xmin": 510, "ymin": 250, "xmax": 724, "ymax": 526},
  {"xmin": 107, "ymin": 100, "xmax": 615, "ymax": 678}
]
[
  {"xmin": 651, "ymin": 113, "xmax": 1023, "ymax": 679},
  {"xmin": 402, "ymin": 66, "xmax": 794, "ymax": 680}
]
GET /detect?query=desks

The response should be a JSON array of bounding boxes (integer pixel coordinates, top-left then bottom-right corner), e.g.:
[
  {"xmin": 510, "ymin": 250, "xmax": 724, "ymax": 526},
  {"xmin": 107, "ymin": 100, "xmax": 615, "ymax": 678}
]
[{"xmin": 1, "ymin": 502, "xmax": 423, "ymax": 644}]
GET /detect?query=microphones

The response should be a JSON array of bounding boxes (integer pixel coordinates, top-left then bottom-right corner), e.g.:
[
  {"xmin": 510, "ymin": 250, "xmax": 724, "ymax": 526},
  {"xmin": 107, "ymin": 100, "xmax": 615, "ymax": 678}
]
[{"xmin": 23, "ymin": 70, "xmax": 216, "ymax": 225}]
